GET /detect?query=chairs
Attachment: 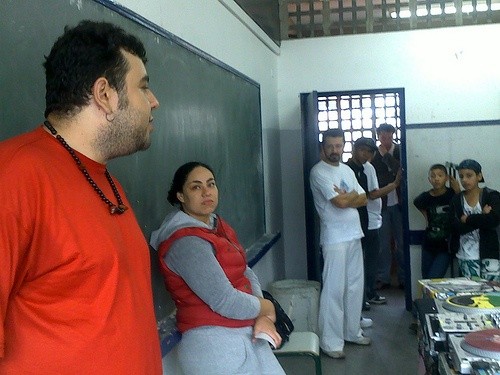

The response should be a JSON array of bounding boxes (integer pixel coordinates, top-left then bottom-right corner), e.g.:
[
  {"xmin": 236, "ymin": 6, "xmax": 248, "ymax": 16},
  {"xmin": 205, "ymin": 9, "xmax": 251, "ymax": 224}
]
[{"xmin": 271, "ymin": 331, "xmax": 322, "ymax": 375}]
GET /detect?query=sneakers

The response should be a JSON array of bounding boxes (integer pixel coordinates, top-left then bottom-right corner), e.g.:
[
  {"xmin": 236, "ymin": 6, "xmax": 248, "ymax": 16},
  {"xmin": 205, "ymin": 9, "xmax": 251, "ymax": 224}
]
[
  {"xmin": 363, "ymin": 301, "xmax": 371, "ymax": 310},
  {"xmin": 321, "ymin": 347, "xmax": 347, "ymax": 359},
  {"xmin": 345, "ymin": 335, "xmax": 371, "ymax": 346},
  {"xmin": 359, "ymin": 316, "xmax": 373, "ymax": 329},
  {"xmin": 370, "ymin": 295, "xmax": 386, "ymax": 305}
]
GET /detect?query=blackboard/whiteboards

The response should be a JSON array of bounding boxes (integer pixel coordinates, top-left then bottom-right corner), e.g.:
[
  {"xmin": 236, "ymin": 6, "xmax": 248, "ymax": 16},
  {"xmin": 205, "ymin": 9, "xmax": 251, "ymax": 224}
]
[{"xmin": 1, "ymin": 0, "xmax": 266, "ymax": 325}]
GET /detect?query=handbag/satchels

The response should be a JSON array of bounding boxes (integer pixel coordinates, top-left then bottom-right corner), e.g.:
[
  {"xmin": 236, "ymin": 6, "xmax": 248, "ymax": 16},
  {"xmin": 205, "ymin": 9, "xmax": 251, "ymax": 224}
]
[{"xmin": 259, "ymin": 290, "xmax": 294, "ymax": 351}]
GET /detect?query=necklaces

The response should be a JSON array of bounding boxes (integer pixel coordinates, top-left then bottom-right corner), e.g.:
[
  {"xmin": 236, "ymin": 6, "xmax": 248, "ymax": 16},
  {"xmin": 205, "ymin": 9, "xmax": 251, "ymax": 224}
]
[{"xmin": 44, "ymin": 120, "xmax": 127, "ymax": 214}]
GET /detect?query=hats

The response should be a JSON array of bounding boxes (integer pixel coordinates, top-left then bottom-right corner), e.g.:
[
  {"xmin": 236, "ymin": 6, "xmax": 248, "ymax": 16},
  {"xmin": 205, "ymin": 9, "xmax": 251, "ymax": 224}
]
[
  {"xmin": 456, "ymin": 159, "xmax": 484, "ymax": 185},
  {"xmin": 354, "ymin": 136, "xmax": 378, "ymax": 151}
]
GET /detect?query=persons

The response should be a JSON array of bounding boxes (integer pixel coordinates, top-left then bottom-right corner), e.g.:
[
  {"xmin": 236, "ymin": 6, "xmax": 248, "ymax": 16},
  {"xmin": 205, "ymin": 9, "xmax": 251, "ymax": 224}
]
[
  {"xmin": 311, "ymin": 130, "xmax": 371, "ymax": 359},
  {"xmin": 446, "ymin": 160, "xmax": 500, "ymax": 280},
  {"xmin": 370, "ymin": 123, "xmax": 404, "ymax": 290},
  {"xmin": 362, "ymin": 150, "xmax": 403, "ymax": 311},
  {"xmin": 149, "ymin": 162, "xmax": 287, "ymax": 375},
  {"xmin": 408, "ymin": 164, "xmax": 461, "ymax": 330},
  {"xmin": 345, "ymin": 137, "xmax": 373, "ymax": 328},
  {"xmin": 0, "ymin": 20, "xmax": 162, "ymax": 375}
]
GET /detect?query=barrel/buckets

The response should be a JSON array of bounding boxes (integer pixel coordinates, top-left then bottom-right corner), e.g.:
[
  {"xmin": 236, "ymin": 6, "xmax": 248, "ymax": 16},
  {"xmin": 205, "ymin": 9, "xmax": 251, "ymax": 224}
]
[{"xmin": 271, "ymin": 278, "xmax": 319, "ymax": 333}]
